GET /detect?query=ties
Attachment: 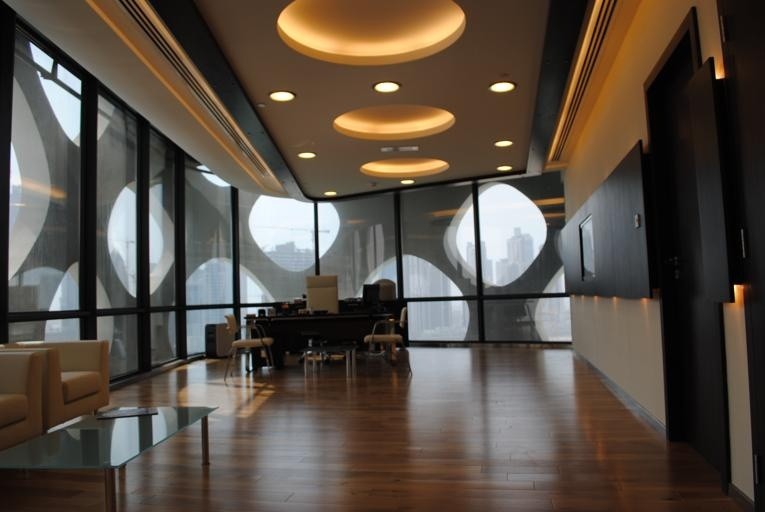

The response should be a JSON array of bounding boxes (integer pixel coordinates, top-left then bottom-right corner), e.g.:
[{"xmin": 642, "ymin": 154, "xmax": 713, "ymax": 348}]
[{"xmin": 579, "ymin": 213, "xmax": 597, "ymax": 282}]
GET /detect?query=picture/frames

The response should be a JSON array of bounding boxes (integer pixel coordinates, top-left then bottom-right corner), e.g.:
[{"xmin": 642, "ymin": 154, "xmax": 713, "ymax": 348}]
[{"xmin": 247, "ymin": 309, "xmax": 391, "ymax": 368}]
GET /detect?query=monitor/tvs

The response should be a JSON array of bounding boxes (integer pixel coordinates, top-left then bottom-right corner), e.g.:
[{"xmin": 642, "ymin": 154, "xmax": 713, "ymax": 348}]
[{"xmin": 95, "ymin": 405, "xmax": 159, "ymax": 419}]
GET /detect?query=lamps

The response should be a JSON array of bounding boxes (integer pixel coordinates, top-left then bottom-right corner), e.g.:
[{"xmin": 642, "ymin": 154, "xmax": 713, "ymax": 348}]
[
  {"xmin": 1, "ymin": 338, "xmax": 114, "ymax": 481},
  {"xmin": 364, "ymin": 305, "xmax": 412, "ymax": 376},
  {"xmin": 223, "ymin": 314, "xmax": 278, "ymax": 389}
]
